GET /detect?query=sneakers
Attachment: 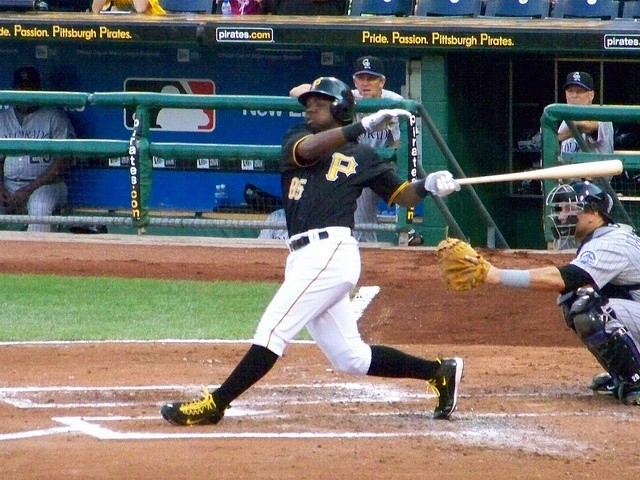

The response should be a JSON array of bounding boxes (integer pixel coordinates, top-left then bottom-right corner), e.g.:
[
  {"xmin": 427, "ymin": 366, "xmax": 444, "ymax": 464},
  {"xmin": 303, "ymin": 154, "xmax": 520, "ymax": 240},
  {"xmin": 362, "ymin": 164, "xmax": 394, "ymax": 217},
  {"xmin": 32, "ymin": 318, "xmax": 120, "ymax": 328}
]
[
  {"xmin": 597, "ymin": 378, "xmax": 615, "ymax": 394},
  {"xmin": 425, "ymin": 354, "xmax": 465, "ymax": 421},
  {"xmin": 159, "ymin": 394, "xmax": 225, "ymax": 429}
]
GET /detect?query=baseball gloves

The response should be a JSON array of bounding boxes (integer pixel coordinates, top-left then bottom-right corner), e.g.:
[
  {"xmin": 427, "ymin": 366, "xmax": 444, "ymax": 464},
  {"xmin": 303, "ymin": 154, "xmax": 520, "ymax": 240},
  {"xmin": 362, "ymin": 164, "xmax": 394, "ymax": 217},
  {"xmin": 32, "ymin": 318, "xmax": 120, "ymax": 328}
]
[{"xmin": 436, "ymin": 236, "xmax": 487, "ymax": 291}]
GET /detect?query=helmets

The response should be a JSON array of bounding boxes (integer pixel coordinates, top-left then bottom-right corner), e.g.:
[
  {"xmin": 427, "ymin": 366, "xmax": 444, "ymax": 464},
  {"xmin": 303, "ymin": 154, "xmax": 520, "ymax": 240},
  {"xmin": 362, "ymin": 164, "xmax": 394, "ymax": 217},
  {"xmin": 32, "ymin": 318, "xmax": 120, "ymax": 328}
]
[
  {"xmin": 299, "ymin": 76, "xmax": 354, "ymax": 121},
  {"xmin": 545, "ymin": 179, "xmax": 613, "ymax": 243}
]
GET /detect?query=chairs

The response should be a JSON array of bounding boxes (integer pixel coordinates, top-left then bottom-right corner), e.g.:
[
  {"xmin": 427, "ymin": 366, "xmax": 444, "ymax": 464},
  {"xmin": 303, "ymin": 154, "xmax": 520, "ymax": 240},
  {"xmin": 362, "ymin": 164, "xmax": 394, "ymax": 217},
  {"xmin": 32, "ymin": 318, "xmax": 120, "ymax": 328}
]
[
  {"xmin": 160, "ymin": 0, "xmax": 211, "ymax": 13},
  {"xmin": 622, "ymin": 0, "xmax": 640, "ymax": 20},
  {"xmin": 552, "ymin": 0, "xmax": 621, "ymax": 19},
  {"xmin": 483, "ymin": 1, "xmax": 551, "ymax": 19},
  {"xmin": 344, "ymin": 0, "xmax": 414, "ymax": 17},
  {"xmin": 414, "ymin": 0, "xmax": 484, "ymax": 17}
]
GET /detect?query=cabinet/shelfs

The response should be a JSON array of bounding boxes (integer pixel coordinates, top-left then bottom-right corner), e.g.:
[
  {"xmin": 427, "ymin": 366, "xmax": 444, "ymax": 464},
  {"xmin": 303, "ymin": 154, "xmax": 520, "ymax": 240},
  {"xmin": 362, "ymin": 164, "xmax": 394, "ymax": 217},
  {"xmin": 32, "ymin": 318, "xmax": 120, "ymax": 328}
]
[{"xmin": 508, "ymin": 55, "xmax": 639, "ymax": 202}]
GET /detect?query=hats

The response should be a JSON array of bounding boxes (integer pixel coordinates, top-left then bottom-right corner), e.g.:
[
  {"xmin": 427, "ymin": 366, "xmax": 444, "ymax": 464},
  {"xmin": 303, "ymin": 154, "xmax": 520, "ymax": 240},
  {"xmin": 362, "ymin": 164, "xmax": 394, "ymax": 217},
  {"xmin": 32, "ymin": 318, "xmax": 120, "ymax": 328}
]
[
  {"xmin": 563, "ymin": 71, "xmax": 594, "ymax": 93},
  {"xmin": 14, "ymin": 66, "xmax": 40, "ymax": 90},
  {"xmin": 352, "ymin": 55, "xmax": 385, "ymax": 81}
]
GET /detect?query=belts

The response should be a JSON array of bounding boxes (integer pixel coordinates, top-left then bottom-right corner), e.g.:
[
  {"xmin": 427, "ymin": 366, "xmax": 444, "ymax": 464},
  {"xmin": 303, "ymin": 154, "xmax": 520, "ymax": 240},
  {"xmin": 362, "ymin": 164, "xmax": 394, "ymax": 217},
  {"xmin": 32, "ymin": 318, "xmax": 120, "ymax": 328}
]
[{"xmin": 287, "ymin": 232, "xmax": 330, "ymax": 253}]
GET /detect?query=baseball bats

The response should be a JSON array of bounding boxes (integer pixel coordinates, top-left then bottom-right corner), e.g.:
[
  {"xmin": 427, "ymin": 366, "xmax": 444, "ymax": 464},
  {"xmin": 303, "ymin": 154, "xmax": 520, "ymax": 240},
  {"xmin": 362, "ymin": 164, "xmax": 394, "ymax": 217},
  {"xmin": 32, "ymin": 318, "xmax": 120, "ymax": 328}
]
[{"xmin": 452, "ymin": 160, "xmax": 624, "ymax": 184}]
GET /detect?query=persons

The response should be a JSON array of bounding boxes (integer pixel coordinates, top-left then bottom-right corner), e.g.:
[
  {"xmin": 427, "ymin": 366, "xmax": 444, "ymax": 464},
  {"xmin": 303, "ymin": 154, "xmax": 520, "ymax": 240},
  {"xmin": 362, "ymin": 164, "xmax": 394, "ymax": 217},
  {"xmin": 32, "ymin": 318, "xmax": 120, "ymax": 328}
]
[
  {"xmin": 539, "ymin": 70, "xmax": 616, "ymax": 237},
  {"xmin": 159, "ymin": 74, "xmax": 464, "ymax": 426},
  {"xmin": 215, "ymin": 0, "xmax": 269, "ymax": 14},
  {"xmin": 1, "ymin": 65, "xmax": 79, "ymax": 233},
  {"xmin": 348, "ymin": 54, "xmax": 424, "ymax": 247},
  {"xmin": 91, "ymin": 0, "xmax": 167, "ymax": 16},
  {"xmin": 437, "ymin": 178, "xmax": 639, "ymax": 404},
  {"xmin": 256, "ymin": 207, "xmax": 288, "ymax": 241}
]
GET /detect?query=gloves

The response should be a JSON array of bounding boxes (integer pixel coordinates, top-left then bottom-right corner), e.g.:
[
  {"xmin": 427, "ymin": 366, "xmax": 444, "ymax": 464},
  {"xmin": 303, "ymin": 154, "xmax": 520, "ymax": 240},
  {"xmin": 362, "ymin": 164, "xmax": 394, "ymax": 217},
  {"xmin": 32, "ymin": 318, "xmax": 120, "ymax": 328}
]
[{"xmin": 425, "ymin": 170, "xmax": 462, "ymax": 198}]
